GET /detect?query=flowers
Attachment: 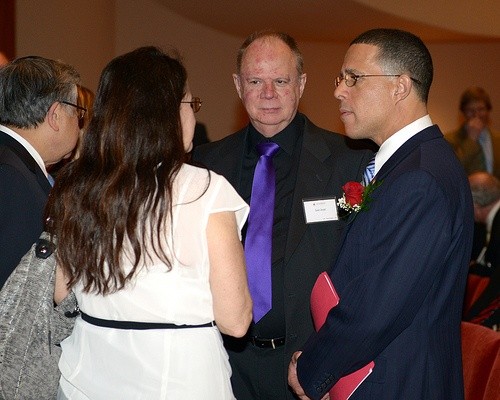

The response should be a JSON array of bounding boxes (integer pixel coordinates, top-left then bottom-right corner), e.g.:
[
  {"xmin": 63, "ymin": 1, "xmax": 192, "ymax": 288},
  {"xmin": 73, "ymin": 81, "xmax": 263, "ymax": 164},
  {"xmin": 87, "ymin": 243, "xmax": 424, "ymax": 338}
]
[{"xmin": 337, "ymin": 178, "xmax": 385, "ymax": 225}]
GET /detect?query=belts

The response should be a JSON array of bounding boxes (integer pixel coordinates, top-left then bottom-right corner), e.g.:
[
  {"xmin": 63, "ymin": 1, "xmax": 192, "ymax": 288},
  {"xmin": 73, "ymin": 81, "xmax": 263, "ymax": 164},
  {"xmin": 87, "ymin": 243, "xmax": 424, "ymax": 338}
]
[{"xmin": 248, "ymin": 336, "xmax": 285, "ymax": 349}]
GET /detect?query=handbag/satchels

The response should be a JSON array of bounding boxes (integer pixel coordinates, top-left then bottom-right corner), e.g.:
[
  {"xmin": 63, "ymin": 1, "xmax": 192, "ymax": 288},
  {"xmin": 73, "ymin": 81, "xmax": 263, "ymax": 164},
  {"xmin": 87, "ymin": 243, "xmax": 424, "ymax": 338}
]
[{"xmin": 1, "ymin": 178, "xmax": 79, "ymax": 400}]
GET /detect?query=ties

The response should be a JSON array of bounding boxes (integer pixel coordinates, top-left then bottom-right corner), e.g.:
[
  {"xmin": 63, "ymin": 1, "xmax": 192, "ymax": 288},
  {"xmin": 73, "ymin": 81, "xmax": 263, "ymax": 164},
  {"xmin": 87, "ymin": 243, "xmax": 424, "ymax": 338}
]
[
  {"xmin": 360, "ymin": 155, "xmax": 376, "ymax": 189},
  {"xmin": 244, "ymin": 142, "xmax": 280, "ymax": 326}
]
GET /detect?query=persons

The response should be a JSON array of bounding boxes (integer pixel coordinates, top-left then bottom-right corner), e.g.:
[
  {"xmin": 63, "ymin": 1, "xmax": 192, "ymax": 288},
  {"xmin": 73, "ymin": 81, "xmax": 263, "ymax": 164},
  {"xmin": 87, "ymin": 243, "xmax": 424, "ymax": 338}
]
[
  {"xmin": 0, "ymin": 54, "xmax": 94, "ymax": 293},
  {"xmin": 468, "ymin": 173, "xmax": 500, "ymax": 332},
  {"xmin": 43, "ymin": 45, "xmax": 254, "ymax": 400},
  {"xmin": 442, "ymin": 85, "xmax": 500, "ymax": 177},
  {"xmin": 279, "ymin": 27, "xmax": 475, "ymax": 400},
  {"xmin": 206, "ymin": 28, "xmax": 378, "ymax": 400}
]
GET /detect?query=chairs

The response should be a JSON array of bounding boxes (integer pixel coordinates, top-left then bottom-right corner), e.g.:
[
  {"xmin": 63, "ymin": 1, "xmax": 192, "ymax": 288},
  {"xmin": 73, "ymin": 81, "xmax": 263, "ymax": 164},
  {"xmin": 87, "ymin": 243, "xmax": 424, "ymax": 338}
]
[{"xmin": 461, "ymin": 320, "xmax": 500, "ymax": 400}]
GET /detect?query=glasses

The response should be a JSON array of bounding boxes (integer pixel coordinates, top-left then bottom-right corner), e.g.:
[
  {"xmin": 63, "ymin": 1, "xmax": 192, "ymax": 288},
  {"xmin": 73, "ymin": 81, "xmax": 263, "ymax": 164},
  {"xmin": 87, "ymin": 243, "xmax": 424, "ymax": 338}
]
[
  {"xmin": 335, "ymin": 73, "xmax": 421, "ymax": 88},
  {"xmin": 63, "ymin": 101, "xmax": 86, "ymax": 119},
  {"xmin": 181, "ymin": 96, "xmax": 202, "ymax": 113}
]
